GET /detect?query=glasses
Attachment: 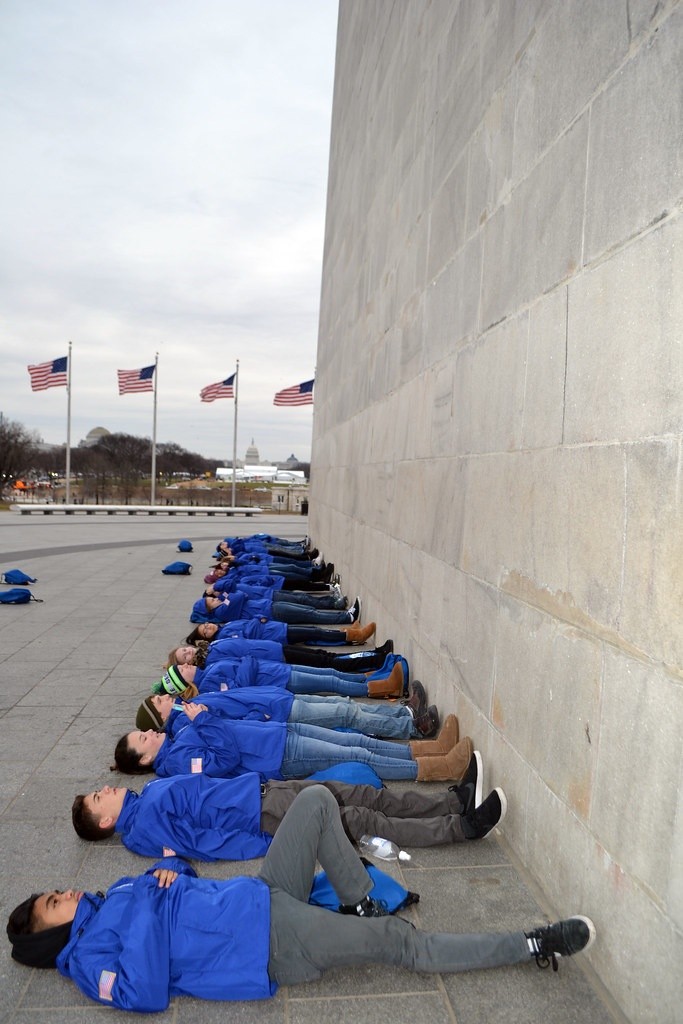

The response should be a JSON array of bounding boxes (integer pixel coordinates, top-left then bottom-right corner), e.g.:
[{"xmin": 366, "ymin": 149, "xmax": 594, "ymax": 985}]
[{"xmin": 203, "ymin": 621, "xmax": 209, "ymax": 639}]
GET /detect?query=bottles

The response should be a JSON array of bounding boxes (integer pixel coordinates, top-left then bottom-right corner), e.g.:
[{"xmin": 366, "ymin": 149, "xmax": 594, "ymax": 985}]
[{"xmin": 359, "ymin": 834, "xmax": 412, "ymax": 862}]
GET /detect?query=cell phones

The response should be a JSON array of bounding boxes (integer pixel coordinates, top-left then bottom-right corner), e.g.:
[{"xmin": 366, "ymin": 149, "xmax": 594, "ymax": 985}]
[{"xmin": 173, "ymin": 704, "xmax": 184, "ymax": 711}]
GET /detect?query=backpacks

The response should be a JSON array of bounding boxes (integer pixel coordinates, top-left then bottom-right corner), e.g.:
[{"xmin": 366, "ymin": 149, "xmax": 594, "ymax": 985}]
[
  {"xmin": 295, "ymin": 761, "xmax": 419, "ymax": 915},
  {"xmin": 178, "ymin": 539, "xmax": 193, "ymax": 552},
  {"xmin": 161, "ymin": 562, "xmax": 193, "ymax": 575},
  {"xmin": 366, "ymin": 653, "xmax": 409, "ymax": 689},
  {"xmin": 0, "ymin": 569, "xmax": 44, "ymax": 604}
]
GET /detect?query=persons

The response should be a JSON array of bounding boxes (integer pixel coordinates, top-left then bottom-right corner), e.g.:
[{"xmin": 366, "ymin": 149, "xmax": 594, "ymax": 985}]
[
  {"xmin": 135, "ymin": 680, "xmax": 439, "ymax": 741},
  {"xmin": 169, "ymin": 638, "xmax": 394, "ymax": 672},
  {"xmin": 153, "ymin": 652, "xmax": 408, "ymax": 698},
  {"xmin": 186, "ymin": 614, "xmax": 375, "ymax": 644},
  {"xmin": 7, "ymin": 785, "xmax": 596, "ymax": 1006},
  {"xmin": 73, "ymin": 750, "xmax": 507, "ymax": 861},
  {"xmin": 190, "ymin": 533, "xmax": 361, "ymax": 624},
  {"xmin": 110, "ymin": 702, "xmax": 472, "ymax": 781}
]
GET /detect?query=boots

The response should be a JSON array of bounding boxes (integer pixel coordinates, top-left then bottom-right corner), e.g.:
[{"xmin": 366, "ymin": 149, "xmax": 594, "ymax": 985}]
[
  {"xmin": 367, "ymin": 660, "xmax": 404, "ymax": 697},
  {"xmin": 408, "ymin": 713, "xmax": 458, "ymax": 761},
  {"xmin": 365, "ymin": 671, "xmax": 380, "ymax": 679},
  {"xmin": 415, "ymin": 736, "xmax": 472, "ymax": 783},
  {"xmin": 339, "ymin": 620, "xmax": 376, "ymax": 644}
]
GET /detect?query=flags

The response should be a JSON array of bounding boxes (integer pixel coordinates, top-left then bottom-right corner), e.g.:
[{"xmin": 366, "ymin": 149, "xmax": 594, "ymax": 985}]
[
  {"xmin": 117, "ymin": 365, "xmax": 155, "ymax": 395},
  {"xmin": 200, "ymin": 372, "xmax": 235, "ymax": 402},
  {"xmin": 27, "ymin": 357, "xmax": 67, "ymax": 391},
  {"xmin": 273, "ymin": 379, "xmax": 315, "ymax": 407}
]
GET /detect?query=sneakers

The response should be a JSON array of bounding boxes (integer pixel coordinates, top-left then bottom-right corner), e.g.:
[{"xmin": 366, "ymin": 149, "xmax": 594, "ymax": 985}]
[
  {"xmin": 346, "ymin": 597, "xmax": 362, "ymax": 624},
  {"xmin": 337, "ymin": 893, "xmax": 416, "ymax": 929},
  {"xmin": 448, "ymin": 750, "xmax": 482, "ymax": 818},
  {"xmin": 461, "ymin": 787, "xmax": 508, "ymax": 839},
  {"xmin": 408, "ymin": 705, "xmax": 439, "ymax": 739},
  {"xmin": 399, "ymin": 680, "xmax": 426, "ymax": 721},
  {"xmin": 524, "ymin": 913, "xmax": 596, "ymax": 972}
]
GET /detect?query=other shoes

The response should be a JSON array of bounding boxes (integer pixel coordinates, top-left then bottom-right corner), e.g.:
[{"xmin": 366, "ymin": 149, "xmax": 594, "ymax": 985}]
[
  {"xmin": 300, "ymin": 535, "xmax": 348, "ymax": 610},
  {"xmin": 367, "ymin": 639, "xmax": 393, "ymax": 669}
]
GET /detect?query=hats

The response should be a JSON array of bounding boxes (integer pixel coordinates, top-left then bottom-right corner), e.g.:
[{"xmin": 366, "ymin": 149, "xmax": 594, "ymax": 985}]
[
  {"xmin": 150, "ymin": 663, "xmax": 189, "ymax": 697},
  {"xmin": 136, "ymin": 699, "xmax": 163, "ymax": 732},
  {"xmin": 204, "ymin": 570, "xmax": 218, "ymax": 584},
  {"xmin": 216, "ymin": 549, "xmax": 228, "ymax": 562}
]
